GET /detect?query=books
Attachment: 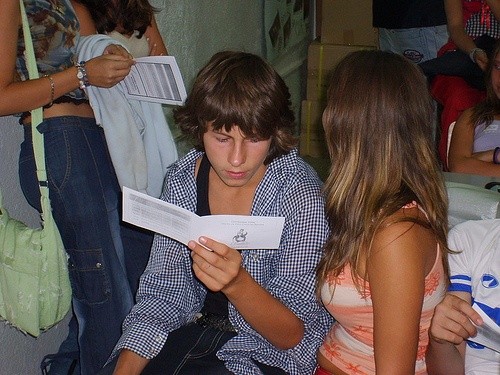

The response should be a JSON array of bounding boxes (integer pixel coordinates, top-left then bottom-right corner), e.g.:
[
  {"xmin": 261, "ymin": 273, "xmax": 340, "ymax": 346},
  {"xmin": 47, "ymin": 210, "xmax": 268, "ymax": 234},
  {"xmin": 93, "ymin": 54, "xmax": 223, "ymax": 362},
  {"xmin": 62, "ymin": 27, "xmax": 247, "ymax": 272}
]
[
  {"xmin": 123, "ymin": 186, "xmax": 286, "ymax": 250},
  {"xmin": 120, "ymin": 56, "xmax": 188, "ymax": 106}
]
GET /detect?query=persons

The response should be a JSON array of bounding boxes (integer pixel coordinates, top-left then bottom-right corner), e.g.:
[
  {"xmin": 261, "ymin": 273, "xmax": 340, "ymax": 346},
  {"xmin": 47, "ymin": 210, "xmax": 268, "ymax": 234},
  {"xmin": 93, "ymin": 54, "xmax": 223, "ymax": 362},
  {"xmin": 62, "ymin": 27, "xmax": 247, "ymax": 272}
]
[
  {"xmin": 448, "ymin": 46, "xmax": 500, "ymax": 177},
  {"xmin": 105, "ymin": 49, "xmax": 336, "ymax": 375},
  {"xmin": 313, "ymin": 47, "xmax": 462, "ymax": 375},
  {"xmin": 444, "ymin": 0, "xmax": 500, "ymax": 71},
  {"xmin": 0, "ymin": 0, "xmax": 178, "ymax": 374},
  {"xmin": 424, "ymin": 217, "xmax": 500, "ymax": 375}
]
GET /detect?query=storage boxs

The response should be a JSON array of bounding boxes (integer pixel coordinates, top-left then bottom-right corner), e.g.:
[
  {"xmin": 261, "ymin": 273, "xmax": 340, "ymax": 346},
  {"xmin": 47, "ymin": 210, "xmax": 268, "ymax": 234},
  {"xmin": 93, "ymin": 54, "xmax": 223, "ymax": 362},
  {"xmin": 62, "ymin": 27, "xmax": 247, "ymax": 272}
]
[{"xmin": 299, "ymin": 0, "xmax": 379, "ymax": 161}]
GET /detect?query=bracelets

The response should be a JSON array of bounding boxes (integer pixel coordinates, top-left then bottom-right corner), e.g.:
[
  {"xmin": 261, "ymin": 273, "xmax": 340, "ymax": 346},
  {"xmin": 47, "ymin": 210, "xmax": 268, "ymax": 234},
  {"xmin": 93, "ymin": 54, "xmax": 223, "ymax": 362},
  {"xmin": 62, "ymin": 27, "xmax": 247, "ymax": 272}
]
[
  {"xmin": 40, "ymin": 75, "xmax": 54, "ymax": 108},
  {"xmin": 469, "ymin": 48, "xmax": 481, "ymax": 63},
  {"xmin": 493, "ymin": 147, "xmax": 500, "ymax": 163},
  {"xmin": 75, "ymin": 60, "xmax": 89, "ymax": 89}
]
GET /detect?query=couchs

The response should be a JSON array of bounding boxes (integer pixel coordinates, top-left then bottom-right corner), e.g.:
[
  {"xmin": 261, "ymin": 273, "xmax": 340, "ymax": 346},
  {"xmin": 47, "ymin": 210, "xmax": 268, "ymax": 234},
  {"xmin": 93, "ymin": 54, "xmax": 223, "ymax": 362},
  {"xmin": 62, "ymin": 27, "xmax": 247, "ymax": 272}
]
[{"xmin": 438, "ymin": 172, "xmax": 500, "ymax": 231}]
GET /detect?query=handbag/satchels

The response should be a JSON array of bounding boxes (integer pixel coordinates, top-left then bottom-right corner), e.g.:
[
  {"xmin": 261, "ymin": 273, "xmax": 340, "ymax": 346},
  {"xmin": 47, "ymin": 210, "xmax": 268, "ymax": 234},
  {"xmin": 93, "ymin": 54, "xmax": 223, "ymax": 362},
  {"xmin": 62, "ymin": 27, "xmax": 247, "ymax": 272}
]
[{"xmin": 0, "ymin": 197, "xmax": 72, "ymax": 336}]
